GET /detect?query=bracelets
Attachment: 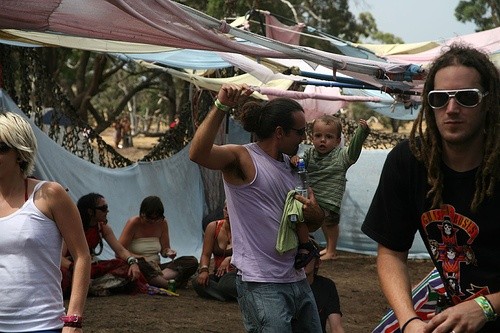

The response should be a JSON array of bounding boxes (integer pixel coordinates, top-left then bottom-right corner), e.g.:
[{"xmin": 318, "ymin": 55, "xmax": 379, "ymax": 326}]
[
  {"xmin": 214, "ymin": 99, "xmax": 231, "ymax": 112},
  {"xmin": 127, "ymin": 256, "xmax": 138, "ymax": 265},
  {"xmin": 401, "ymin": 316, "xmax": 423, "ymax": 333},
  {"xmin": 199, "ymin": 265, "xmax": 209, "ymax": 273},
  {"xmin": 162, "ymin": 248, "xmax": 169, "ymax": 257},
  {"xmin": 60, "ymin": 316, "xmax": 82, "ymax": 328},
  {"xmin": 474, "ymin": 294, "xmax": 498, "ymax": 322}
]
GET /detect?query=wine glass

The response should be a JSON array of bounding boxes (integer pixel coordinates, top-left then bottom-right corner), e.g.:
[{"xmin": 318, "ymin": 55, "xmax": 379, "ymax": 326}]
[{"xmin": 168, "ymin": 248, "xmax": 176, "ymax": 264}]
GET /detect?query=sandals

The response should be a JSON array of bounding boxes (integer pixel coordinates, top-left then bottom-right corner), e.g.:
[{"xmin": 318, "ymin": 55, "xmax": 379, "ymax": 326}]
[{"xmin": 294, "ymin": 239, "xmax": 317, "ymax": 269}]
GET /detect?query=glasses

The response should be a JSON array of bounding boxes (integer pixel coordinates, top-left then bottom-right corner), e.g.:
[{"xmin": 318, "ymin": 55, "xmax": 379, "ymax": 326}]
[
  {"xmin": 291, "ymin": 127, "xmax": 306, "ymax": 136},
  {"xmin": 95, "ymin": 204, "xmax": 110, "ymax": 213},
  {"xmin": 0, "ymin": 142, "xmax": 10, "ymax": 153},
  {"xmin": 426, "ymin": 89, "xmax": 487, "ymax": 110}
]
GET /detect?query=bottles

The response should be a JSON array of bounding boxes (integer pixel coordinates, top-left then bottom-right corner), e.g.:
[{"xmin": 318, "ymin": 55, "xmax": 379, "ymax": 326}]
[
  {"xmin": 436, "ymin": 293, "xmax": 448, "ymax": 316},
  {"xmin": 169, "ymin": 279, "xmax": 176, "ymax": 294},
  {"xmin": 295, "ymin": 160, "xmax": 310, "ymax": 200}
]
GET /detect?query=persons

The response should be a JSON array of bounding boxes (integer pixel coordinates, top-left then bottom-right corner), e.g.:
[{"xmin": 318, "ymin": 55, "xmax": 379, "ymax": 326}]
[
  {"xmin": 61, "ymin": 193, "xmax": 140, "ymax": 300},
  {"xmin": 169, "ymin": 114, "xmax": 180, "ymax": 129},
  {"xmin": 115, "ymin": 196, "xmax": 199, "ymax": 293},
  {"xmin": 192, "ymin": 200, "xmax": 239, "ymax": 302},
  {"xmin": 362, "ymin": 39, "xmax": 500, "ymax": 333},
  {"xmin": 290, "ymin": 113, "xmax": 369, "ymax": 261},
  {"xmin": 301, "ymin": 234, "xmax": 345, "ymax": 333},
  {"xmin": 0, "ymin": 111, "xmax": 91, "ymax": 333},
  {"xmin": 189, "ymin": 82, "xmax": 325, "ymax": 333},
  {"xmin": 113, "ymin": 115, "xmax": 131, "ymax": 150}
]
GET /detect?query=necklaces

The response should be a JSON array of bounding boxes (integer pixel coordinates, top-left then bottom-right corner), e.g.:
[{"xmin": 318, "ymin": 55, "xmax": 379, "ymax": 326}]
[
  {"xmin": 84, "ymin": 220, "xmax": 104, "ymax": 256},
  {"xmin": 225, "ymin": 221, "xmax": 231, "ymax": 244}
]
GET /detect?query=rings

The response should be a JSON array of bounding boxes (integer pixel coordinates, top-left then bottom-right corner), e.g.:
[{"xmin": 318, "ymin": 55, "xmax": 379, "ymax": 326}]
[
  {"xmin": 303, "ymin": 204, "xmax": 308, "ymax": 208},
  {"xmin": 450, "ymin": 330, "xmax": 455, "ymax": 333},
  {"xmin": 222, "ymin": 266, "xmax": 225, "ymax": 270}
]
